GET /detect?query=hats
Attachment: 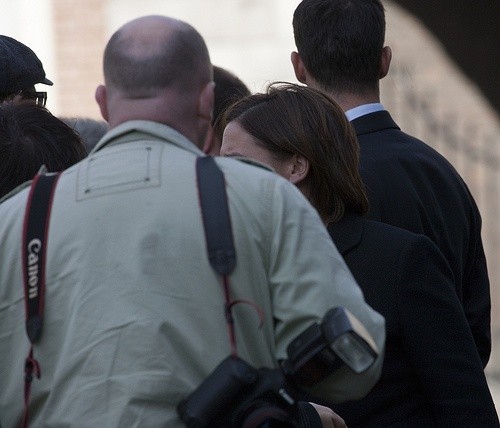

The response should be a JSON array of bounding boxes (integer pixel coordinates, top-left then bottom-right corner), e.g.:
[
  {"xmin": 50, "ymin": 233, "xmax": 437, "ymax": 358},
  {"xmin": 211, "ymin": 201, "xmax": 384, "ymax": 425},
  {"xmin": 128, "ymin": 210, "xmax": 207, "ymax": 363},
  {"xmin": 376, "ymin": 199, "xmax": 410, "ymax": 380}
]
[{"xmin": 0, "ymin": 35, "xmax": 53, "ymax": 88}]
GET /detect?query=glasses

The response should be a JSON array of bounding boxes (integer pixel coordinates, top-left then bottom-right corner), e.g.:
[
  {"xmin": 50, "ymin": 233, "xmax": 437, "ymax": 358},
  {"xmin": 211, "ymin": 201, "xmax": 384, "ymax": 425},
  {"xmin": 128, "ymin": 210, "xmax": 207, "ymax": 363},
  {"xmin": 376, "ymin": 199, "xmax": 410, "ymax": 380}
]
[{"xmin": 23, "ymin": 92, "xmax": 48, "ymax": 108}]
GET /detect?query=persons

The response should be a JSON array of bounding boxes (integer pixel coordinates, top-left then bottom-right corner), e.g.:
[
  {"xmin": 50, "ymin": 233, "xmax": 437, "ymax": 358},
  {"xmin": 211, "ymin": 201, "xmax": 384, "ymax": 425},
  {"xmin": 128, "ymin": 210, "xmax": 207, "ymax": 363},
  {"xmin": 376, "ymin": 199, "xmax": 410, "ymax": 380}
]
[
  {"xmin": 0, "ymin": 14, "xmax": 500, "ymax": 428},
  {"xmin": 290, "ymin": 0, "xmax": 492, "ymax": 370}
]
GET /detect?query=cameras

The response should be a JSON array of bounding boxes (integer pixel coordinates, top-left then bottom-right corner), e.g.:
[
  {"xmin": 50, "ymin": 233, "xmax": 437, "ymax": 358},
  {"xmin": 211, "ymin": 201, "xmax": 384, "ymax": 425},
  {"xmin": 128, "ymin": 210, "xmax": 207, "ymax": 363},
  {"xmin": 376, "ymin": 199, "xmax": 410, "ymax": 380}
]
[{"xmin": 176, "ymin": 305, "xmax": 378, "ymax": 428}]
